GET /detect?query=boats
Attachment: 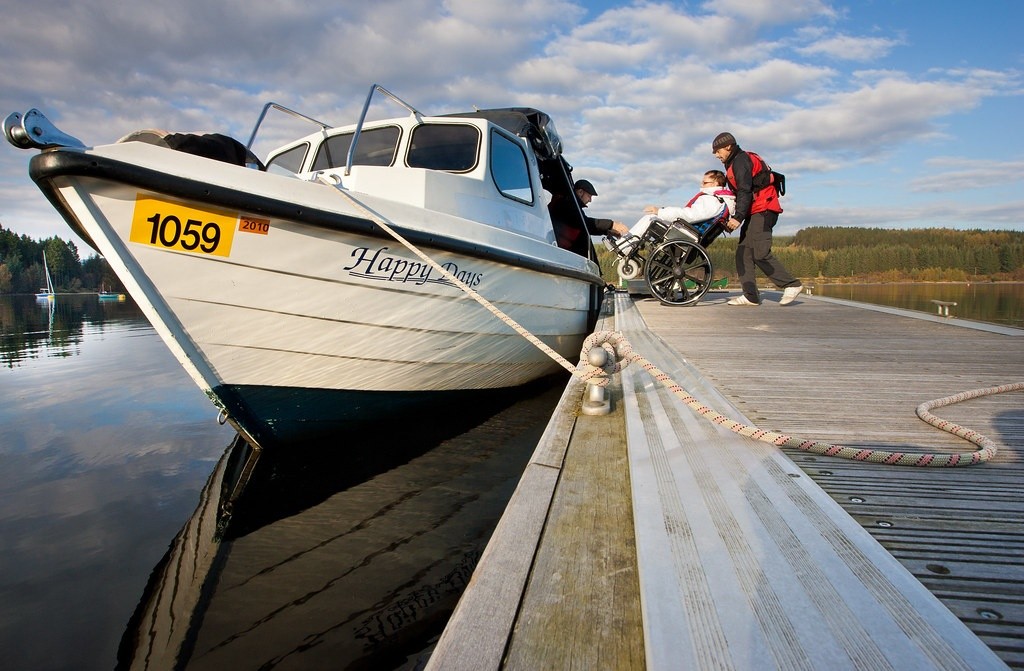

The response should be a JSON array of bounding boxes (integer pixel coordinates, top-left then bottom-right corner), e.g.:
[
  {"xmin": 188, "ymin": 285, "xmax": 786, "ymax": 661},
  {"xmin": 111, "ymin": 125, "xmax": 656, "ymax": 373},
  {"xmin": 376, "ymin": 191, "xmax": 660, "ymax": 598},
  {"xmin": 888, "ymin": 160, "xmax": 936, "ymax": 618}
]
[
  {"xmin": 2, "ymin": 83, "xmax": 605, "ymax": 452},
  {"xmin": 113, "ymin": 401, "xmax": 557, "ymax": 671},
  {"xmin": 97, "ymin": 282, "xmax": 125, "ymax": 298},
  {"xmin": 99, "ymin": 298, "xmax": 125, "ymax": 308}
]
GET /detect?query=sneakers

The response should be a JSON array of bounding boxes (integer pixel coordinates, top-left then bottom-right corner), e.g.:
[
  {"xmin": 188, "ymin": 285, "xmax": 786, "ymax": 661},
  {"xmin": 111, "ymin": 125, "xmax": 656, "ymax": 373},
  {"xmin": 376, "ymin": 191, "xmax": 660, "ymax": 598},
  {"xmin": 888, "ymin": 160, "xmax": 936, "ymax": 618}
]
[
  {"xmin": 727, "ymin": 294, "xmax": 759, "ymax": 306},
  {"xmin": 779, "ymin": 284, "xmax": 804, "ymax": 306}
]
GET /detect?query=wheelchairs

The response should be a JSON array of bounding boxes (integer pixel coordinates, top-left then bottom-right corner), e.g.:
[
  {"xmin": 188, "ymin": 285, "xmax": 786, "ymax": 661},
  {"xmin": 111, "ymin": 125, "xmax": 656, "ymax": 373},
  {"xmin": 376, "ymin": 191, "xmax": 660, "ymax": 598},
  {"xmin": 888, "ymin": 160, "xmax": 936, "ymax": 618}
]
[{"xmin": 600, "ymin": 216, "xmax": 734, "ymax": 307}]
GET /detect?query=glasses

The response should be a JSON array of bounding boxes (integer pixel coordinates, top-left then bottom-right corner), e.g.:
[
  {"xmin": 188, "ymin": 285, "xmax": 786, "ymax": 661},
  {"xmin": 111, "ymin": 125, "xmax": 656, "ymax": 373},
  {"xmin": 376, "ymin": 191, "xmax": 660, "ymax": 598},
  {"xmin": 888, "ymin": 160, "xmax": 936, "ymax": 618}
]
[{"xmin": 701, "ymin": 181, "xmax": 715, "ymax": 185}]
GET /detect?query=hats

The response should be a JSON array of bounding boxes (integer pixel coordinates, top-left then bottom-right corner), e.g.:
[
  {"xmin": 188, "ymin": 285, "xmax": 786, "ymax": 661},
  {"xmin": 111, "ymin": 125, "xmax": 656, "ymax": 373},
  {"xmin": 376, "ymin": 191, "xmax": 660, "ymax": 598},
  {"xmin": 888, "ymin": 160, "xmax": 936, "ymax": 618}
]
[
  {"xmin": 712, "ymin": 132, "xmax": 736, "ymax": 154},
  {"xmin": 573, "ymin": 180, "xmax": 598, "ymax": 196}
]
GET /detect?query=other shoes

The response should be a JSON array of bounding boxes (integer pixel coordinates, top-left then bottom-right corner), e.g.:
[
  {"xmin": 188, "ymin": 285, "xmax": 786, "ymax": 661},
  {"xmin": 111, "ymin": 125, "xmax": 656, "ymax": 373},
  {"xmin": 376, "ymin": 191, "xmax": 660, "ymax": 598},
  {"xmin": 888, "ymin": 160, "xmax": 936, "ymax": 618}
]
[{"xmin": 606, "ymin": 234, "xmax": 626, "ymax": 257}]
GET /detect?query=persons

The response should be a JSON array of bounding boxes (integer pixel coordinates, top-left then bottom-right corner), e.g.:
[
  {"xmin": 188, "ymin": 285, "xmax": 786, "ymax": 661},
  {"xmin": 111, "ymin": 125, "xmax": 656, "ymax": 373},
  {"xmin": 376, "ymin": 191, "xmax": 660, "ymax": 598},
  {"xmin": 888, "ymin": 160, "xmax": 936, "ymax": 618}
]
[
  {"xmin": 712, "ymin": 132, "xmax": 804, "ymax": 305},
  {"xmin": 573, "ymin": 180, "xmax": 629, "ymax": 235},
  {"xmin": 608, "ymin": 170, "xmax": 736, "ymax": 258}
]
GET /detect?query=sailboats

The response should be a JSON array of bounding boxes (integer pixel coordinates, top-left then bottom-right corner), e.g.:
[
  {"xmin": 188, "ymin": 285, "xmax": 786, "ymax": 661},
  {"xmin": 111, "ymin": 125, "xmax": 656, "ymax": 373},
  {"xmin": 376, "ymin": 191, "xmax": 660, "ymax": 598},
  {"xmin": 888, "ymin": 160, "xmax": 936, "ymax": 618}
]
[
  {"xmin": 34, "ymin": 250, "xmax": 56, "ymax": 297},
  {"xmin": 35, "ymin": 297, "xmax": 56, "ymax": 346}
]
